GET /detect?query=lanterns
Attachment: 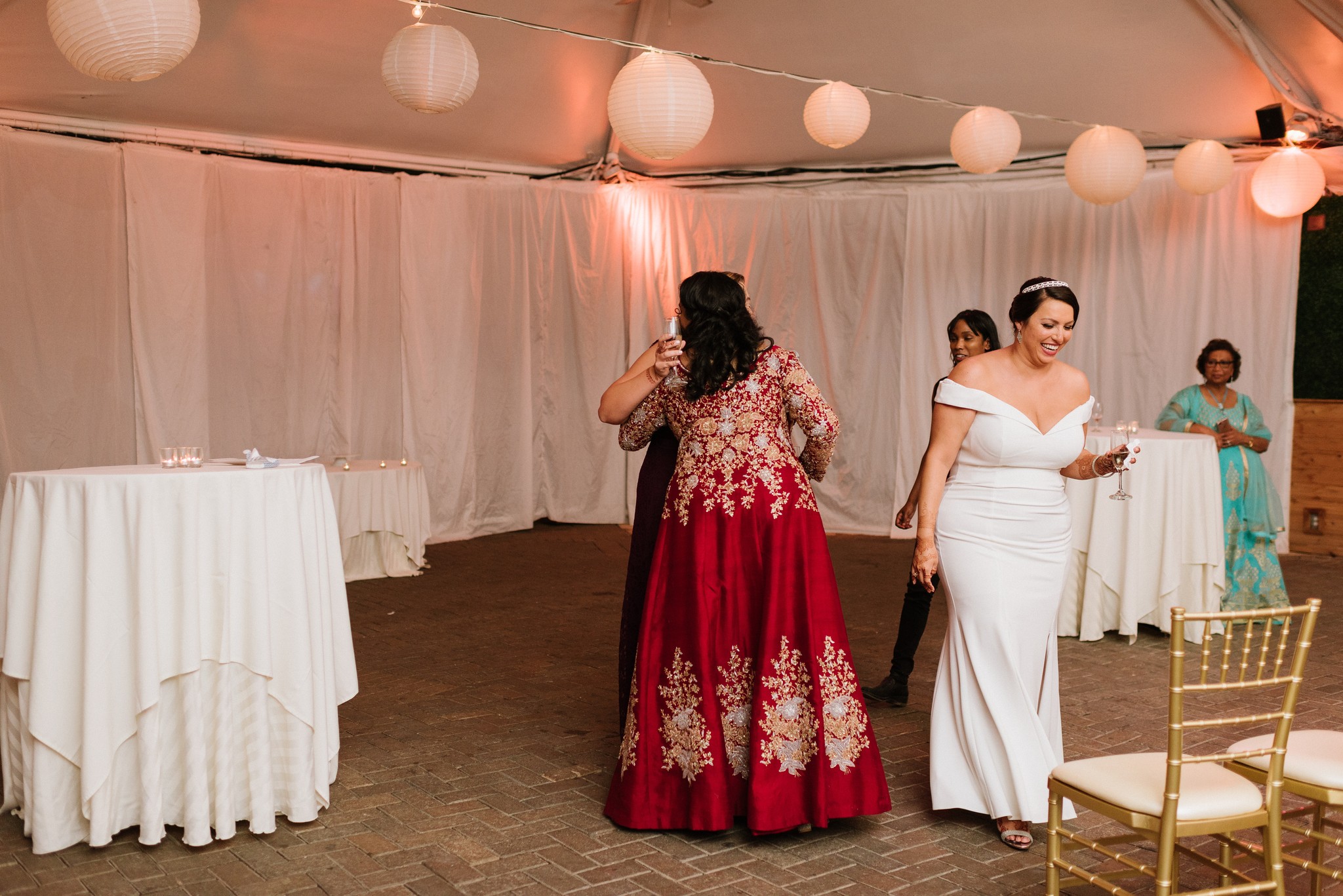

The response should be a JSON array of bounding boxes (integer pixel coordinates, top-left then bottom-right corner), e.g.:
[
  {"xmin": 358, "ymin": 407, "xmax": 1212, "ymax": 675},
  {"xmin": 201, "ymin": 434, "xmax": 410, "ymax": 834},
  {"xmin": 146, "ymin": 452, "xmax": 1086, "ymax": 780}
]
[
  {"xmin": 606, "ymin": 51, "xmax": 714, "ymax": 162},
  {"xmin": 382, "ymin": 22, "xmax": 480, "ymax": 116},
  {"xmin": 1064, "ymin": 125, "xmax": 1147, "ymax": 205},
  {"xmin": 1173, "ymin": 140, "xmax": 1233, "ymax": 194},
  {"xmin": 803, "ymin": 81, "xmax": 871, "ymax": 150},
  {"xmin": 950, "ymin": 106, "xmax": 1022, "ymax": 175},
  {"xmin": 46, "ymin": 0, "xmax": 202, "ymax": 81},
  {"xmin": 1250, "ymin": 149, "xmax": 1325, "ymax": 218}
]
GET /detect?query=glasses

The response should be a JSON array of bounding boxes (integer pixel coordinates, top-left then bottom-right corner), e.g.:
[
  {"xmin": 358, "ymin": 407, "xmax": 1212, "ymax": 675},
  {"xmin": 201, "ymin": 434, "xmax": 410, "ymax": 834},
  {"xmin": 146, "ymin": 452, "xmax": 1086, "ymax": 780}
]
[{"xmin": 1206, "ymin": 360, "xmax": 1233, "ymax": 368}]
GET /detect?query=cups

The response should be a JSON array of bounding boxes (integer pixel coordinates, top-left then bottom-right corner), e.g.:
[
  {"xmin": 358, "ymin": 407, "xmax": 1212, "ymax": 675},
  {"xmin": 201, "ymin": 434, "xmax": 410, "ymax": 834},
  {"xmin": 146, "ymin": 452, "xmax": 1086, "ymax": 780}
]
[
  {"xmin": 187, "ymin": 447, "xmax": 204, "ymax": 468},
  {"xmin": 1116, "ymin": 420, "xmax": 1127, "ymax": 431},
  {"xmin": 176, "ymin": 448, "xmax": 191, "ymax": 468},
  {"xmin": 1128, "ymin": 421, "xmax": 1140, "ymax": 435},
  {"xmin": 159, "ymin": 448, "xmax": 177, "ymax": 468}
]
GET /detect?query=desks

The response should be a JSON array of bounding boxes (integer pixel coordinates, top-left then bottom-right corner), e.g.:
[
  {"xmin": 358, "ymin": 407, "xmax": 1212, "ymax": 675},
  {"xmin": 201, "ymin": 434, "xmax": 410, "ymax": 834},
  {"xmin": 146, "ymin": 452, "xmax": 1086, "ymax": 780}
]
[
  {"xmin": 1057, "ymin": 427, "xmax": 1225, "ymax": 644},
  {"xmin": 325, "ymin": 459, "xmax": 432, "ymax": 581},
  {"xmin": 0, "ymin": 462, "xmax": 359, "ymax": 854}
]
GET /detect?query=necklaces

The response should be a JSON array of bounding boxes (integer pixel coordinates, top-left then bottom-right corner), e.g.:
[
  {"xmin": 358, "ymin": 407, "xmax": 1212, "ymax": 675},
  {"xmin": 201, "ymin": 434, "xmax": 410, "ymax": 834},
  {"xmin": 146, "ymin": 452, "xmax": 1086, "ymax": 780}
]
[{"xmin": 1204, "ymin": 383, "xmax": 1228, "ymax": 414}]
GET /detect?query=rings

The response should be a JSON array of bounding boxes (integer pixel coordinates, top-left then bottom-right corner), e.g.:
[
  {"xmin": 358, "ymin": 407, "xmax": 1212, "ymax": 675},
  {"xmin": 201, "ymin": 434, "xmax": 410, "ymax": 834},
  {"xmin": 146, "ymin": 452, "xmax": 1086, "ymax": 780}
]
[
  {"xmin": 1227, "ymin": 440, "xmax": 1230, "ymax": 444},
  {"xmin": 913, "ymin": 572, "xmax": 919, "ymax": 577}
]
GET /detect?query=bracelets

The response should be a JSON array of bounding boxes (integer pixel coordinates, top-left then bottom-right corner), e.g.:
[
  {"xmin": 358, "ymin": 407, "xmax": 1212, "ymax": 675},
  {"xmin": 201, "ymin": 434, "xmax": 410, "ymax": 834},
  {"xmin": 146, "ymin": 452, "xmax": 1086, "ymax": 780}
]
[
  {"xmin": 1246, "ymin": 435, "xmax": 1254, "ymax": 448},
  {"xmin": 1092, "ymin": 454, "xmax": 1115, "ymax": 478}
]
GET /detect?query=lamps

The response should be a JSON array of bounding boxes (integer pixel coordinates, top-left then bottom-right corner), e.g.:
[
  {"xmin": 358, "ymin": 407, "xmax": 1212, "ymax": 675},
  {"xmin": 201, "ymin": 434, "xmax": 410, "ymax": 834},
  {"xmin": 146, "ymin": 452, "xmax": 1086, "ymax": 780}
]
[{"xmin": 1286, "ymin": 107, "xmax": 1310, "ymax": 142}]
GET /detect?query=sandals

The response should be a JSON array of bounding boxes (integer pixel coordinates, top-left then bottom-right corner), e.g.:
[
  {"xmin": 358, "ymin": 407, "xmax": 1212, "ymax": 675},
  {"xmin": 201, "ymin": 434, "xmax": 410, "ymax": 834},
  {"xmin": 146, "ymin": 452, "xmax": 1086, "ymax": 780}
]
[{"xmin": 997, "ymin": 817, "xmax": 1034, "ymax": 850}]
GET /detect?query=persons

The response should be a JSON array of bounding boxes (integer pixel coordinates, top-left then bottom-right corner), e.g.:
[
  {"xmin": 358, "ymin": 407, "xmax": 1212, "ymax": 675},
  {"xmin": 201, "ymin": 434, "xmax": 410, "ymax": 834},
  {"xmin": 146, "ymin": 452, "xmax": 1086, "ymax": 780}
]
[
  {"xmin": 596, "ymin": 272, "xmax": 752, "ymax": 744},
  {"xmin": 862, "ymin": 310, "xmax": 1003, "ymax": 706},
  {"xmin": 911, "ymin": 277, "xmax": 1141, "ymax": 851},
  {"xmin": 603, "ymin": 270, "xmax": 893, "ymax": 835},
  {"xmin": 1155, "ymin": 339, "xmax": 1293, "ymax": 625}
]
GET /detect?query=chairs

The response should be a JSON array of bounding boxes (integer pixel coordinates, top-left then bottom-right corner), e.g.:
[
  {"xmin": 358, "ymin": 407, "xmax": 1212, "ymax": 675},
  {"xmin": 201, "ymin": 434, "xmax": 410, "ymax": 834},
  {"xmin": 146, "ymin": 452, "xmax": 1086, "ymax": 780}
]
[
  {"xmin": 1045, "ymin": 599, "xmax": 1321, "ymax": 896},
  {"xmin": 1218, "ymin": 730, "xmax": 1343, "ymax": 896}
]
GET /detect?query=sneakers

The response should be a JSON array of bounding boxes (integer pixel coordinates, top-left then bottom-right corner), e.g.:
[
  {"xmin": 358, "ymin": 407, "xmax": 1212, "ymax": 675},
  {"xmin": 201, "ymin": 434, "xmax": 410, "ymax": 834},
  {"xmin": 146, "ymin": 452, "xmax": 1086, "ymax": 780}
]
[{"xmin": 860, "ymin": 675, "xmax": 909, "ymax": 706}]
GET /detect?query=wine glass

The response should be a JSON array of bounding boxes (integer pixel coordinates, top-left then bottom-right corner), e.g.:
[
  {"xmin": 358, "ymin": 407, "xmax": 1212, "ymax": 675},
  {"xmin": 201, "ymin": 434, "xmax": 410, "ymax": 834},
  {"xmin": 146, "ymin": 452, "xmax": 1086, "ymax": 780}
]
[
  {"xmin": 1109, "ymin": 430, "xmax": 1133, "ymax": 500},
  {"xmin": 662, "ymin": 316, "xmax": 688, "ymax": 387},
  {"xmin": 1092, "ymin": 403, "xmax": 1103, "ymax": 433}
]
